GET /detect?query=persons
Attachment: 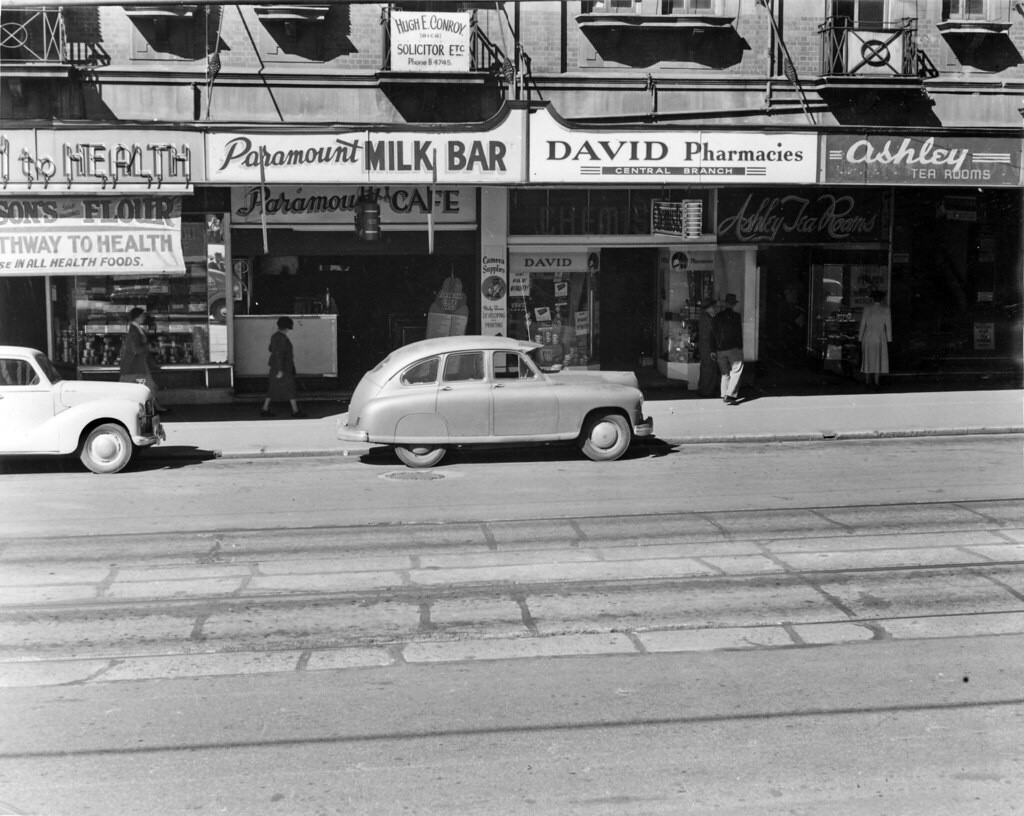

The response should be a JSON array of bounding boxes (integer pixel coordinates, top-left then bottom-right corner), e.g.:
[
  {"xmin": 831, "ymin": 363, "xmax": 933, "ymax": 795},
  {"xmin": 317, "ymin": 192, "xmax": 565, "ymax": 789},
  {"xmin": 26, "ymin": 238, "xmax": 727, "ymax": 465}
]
[
  {"xmin": 260, "ymin": 317, "xmax": 307, "ymax": 417},
  {"xmin": 857, "ymin": 291, "xmax": 892, "ymax": 386},
  {"xmin": 698, "ymin": 293, "xmax": 746, "ymax": 403},
  {"xmin": 118, "ymin": 308, "xmax": 171, "ymax": 413}
]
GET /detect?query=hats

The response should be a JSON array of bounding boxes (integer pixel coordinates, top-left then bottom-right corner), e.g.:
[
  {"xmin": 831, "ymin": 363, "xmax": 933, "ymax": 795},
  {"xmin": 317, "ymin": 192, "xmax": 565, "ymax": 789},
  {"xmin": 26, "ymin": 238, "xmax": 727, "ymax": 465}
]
[
  {"xmin": 869, "ymin": 290, "xmax": 886, "ymax": 298},
  {"xmin": 722, "ymin": 294, "xmax": 739, "ymax": 303},
  {"xmin": 699, "ymin": 297, "xmax": 718, "ymax": 310}
]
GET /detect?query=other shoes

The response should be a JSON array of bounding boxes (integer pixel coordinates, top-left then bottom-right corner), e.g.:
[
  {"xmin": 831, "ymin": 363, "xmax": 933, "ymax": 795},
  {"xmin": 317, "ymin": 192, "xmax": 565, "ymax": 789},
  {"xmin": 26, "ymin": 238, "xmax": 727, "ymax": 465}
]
[
  {"xmin": 260, "ymin": 408, "xmax": 276, "ymax": 417},
  {"xmin": 724, "ymin": 396, "xmax": 738, "ymax": 405},
  {"xmin": 157, "ymin": 407, "xmax": 172, "ymax": 415},
  {"xmin": 291, "ymin": 411, "xmax": 308, "ymax": 418}
]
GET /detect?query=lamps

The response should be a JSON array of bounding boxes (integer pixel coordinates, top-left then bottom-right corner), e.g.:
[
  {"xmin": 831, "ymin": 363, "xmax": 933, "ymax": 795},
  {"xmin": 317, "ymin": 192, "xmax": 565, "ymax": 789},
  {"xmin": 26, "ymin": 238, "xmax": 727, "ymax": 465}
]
[{"xmin": 355, "ymin": 186, "xmax": 382, "ymax": 242}]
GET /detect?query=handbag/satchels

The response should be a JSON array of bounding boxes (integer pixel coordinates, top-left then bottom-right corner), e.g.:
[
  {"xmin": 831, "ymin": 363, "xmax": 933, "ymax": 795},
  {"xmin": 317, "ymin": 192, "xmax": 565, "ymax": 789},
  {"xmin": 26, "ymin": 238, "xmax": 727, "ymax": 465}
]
[
  {"xmin": 120, "ymin": 350, "xmax": 144, "ymax": 373},
  {"xmin": 268, "ymin": 374, "xmax": 280, "ymax": 396}
]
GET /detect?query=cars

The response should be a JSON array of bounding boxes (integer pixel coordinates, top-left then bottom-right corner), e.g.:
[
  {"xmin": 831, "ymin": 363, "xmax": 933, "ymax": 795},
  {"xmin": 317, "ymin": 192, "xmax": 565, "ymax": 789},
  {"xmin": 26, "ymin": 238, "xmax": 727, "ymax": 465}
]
[
  {"xmin": 337, "ymin": 335, "xmax": 654, "ymax": 470},
  {"xmin": 824, "ymin": 277, "xmax": 843, "ymax": 311},
  {"xmin": 208, "ymin": 253, "xmax": 226, "ymax": 324}
]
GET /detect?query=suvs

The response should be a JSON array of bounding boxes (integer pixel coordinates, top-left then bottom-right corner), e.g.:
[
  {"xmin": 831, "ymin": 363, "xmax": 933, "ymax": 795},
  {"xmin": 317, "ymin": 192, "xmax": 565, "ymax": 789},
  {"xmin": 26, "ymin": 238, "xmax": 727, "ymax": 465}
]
[{"xmin": 0, "ymin": 345, "xmax": 167, "ymax": 474}]
